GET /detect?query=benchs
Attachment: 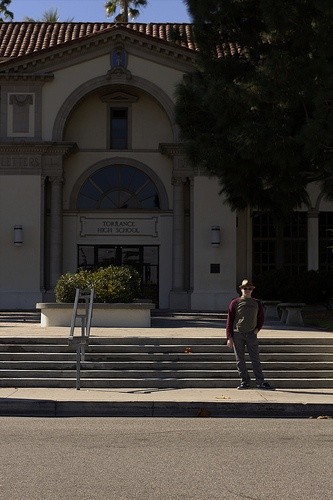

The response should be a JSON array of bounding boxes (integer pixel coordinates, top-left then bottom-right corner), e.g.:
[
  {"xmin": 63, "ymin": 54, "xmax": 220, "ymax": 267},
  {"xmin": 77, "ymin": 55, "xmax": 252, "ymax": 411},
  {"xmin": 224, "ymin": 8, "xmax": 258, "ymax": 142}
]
[
  {"xmin": 278, "ymin": 302, "xmax": 306, "ymax": 325},
  {"xmin": 260, "ymin": 300, "xmax": 280, "ymax": 321}
]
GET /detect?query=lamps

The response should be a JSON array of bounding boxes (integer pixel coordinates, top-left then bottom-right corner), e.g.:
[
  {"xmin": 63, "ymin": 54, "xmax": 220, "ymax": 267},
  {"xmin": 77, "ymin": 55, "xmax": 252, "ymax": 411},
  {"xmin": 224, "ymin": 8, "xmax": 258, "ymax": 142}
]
[
  {"xmin": 13, "ymin": 224, "xmax": 24, "ymax": 247},
  {"xmin": 209, "ymin": 225, "xmax": 223, "ymax": 248}
]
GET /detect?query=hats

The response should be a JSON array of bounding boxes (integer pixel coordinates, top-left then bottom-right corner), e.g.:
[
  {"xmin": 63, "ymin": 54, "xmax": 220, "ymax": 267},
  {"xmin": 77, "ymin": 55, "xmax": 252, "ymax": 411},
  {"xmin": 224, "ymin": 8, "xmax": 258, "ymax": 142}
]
[{"xmin": 239, "ymin": 279, "xmax": 255, "ymax": 288}]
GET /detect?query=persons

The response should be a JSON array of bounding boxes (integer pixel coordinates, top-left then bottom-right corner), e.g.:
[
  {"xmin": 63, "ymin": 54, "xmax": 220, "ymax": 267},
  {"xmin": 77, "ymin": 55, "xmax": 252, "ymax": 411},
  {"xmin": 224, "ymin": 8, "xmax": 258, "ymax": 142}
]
[{"xmin": 226, "ymin": 280, "xmax": 272, "ymax": 390}]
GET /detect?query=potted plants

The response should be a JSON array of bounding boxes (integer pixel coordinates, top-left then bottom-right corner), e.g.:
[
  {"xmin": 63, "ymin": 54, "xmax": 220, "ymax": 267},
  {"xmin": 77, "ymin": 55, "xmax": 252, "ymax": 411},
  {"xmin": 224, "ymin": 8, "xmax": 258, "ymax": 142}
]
[{"xmin": 33, "ymin": 262, "xmax": 156, "ymax": 327}]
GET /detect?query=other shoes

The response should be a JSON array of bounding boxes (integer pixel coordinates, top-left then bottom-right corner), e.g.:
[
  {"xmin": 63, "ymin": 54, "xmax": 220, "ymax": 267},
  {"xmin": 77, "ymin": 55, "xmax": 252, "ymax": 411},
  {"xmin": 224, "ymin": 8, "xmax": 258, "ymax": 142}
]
[
  {"xmin": 258, "ymin": 383, "xmax": 275, "ymax": 391},
  {"xmin": 237, "ymin": 383, "xmax": 251, "ymax": 389}
]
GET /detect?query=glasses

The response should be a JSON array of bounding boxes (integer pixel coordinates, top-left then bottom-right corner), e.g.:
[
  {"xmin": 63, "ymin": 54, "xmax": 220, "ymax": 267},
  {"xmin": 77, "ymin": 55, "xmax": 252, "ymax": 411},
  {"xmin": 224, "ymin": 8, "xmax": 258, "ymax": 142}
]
[{"xmin": 241, "ymin": 287, "xmax": 253, "ymax": 290}]
